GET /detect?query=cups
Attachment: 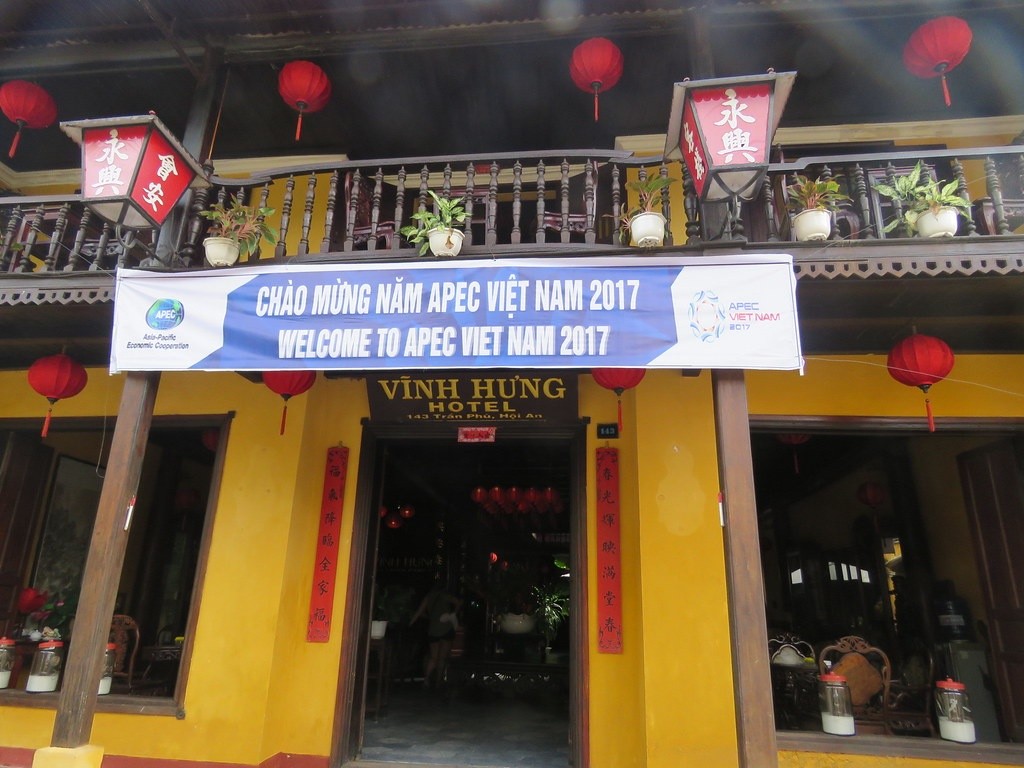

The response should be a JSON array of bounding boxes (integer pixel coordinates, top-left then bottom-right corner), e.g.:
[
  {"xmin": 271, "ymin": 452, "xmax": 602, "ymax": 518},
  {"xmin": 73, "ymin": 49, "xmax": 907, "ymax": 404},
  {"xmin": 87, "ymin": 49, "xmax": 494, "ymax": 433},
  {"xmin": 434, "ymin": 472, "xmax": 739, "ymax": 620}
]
[{"xmin": 883, "ymin": 680, "xmax": 932, "ymax": 740}]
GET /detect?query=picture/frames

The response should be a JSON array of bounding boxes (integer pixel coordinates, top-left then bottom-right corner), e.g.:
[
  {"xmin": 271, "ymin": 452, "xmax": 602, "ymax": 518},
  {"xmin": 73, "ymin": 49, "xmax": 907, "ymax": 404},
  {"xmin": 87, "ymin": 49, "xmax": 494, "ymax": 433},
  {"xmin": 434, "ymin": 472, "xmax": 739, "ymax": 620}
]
[{"xmin": 20, "ymin": 454, "xmax": 158, "ymax": 644}]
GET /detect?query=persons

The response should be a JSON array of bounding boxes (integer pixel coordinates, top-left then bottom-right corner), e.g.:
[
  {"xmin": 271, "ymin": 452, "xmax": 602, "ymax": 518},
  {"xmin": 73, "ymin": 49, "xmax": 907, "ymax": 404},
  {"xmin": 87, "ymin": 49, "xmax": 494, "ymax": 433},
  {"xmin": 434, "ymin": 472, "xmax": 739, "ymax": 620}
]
[
  {"xmin": 408, "ymin": 576, "xmax": 461, "ymax": 689},
  {"xmin": 892, "ymin": 575, "xmax": 917, "ymax": 632}
]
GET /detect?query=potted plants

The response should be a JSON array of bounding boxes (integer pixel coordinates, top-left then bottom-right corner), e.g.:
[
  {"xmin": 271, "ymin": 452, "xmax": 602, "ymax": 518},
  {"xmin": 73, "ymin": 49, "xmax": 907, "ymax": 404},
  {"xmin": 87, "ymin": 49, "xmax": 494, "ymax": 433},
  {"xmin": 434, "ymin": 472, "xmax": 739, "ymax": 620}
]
[
  {"xmin": 371, "ymin": 587, "xmax": 413, "ymax": 640},
  {"xmin": 781, "ymin": 171, "xmax": 855, "ymax": 241},
  {"xmin": 198, "ymin": 192, "xmax": 279, "ymax": 268},
  {"xmin": 602, "ymin": 175, "xmax": 677, "ymax": 249},
  {"xmin": 871, "ymin": 162, "xmax": 975, "ymax": 239},
  {"xmin": 461, "ymin": 566, "xmax": 573, "ymax": 664},
  {"xmin": 398, "ymin": 189, "xmax": 475, "ymax": 258}
]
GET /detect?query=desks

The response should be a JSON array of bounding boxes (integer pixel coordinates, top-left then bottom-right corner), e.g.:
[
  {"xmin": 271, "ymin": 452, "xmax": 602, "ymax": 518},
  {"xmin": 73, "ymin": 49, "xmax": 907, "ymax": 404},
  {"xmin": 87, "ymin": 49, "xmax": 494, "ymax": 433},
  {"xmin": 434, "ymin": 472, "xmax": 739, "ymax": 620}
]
[
  {"xmin": 369, "ymin": 639, "xmax": 398, "ymax": 722},
  {"xmin": 853, "ymin": 164, "xmax": 941, "ymax": 239},
  {"xmin": 416, "ymin": 187, "xmax": 490, "ymax": 246},
  {"xmin": 143, "ymin": 644, "xmax": 180, "ymax": 696}
]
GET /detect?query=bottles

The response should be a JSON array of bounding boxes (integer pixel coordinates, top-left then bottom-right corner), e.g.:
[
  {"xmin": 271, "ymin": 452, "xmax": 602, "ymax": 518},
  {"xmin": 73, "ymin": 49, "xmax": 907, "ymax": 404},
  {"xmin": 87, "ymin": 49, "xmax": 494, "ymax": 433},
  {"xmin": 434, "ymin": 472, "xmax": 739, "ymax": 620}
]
[
  {"xmin": 936, "ymin": 601, "xmax": 999, "ymax": 743},
  {"xmin": 0, "ymin": 637, "xmax": 24, "ymax": 688},
  {"xmin": 94, "ymin": 642, "xmax": 117, "ymax": 694},
  {"xmin": 816, "ymin": 671, "xmax": 856, "ymax": 736},
  {"xmin": 25, "ymin": 639, "xmax": 65, "ymax": 692},
  {"xmin": 31, "ymin": 628, "xmax": 42, "ymax": 641},
  {"xmin": 932, "ymin": 677, "xmax": 977, "ymax": 743}
]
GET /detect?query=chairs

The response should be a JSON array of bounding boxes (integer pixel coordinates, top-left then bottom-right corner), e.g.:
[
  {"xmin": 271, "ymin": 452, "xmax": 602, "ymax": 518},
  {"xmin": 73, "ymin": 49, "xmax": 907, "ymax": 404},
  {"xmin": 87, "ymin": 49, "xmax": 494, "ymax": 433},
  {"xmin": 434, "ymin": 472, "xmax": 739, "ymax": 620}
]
[
  {"xmin": 21, "ymin": 190, "xmax": 126, "ymax": 269},
  {"xmin": 106, "ymin": 616, "xmax": 186, "ymax": 696},
  {"xmin": 340, "ymin": 171, "xmax": 411, "ymax": 250},
  {"xmin": 527, "ymin": 157, "xmax": 598, "ymax": 241},
  {"xmin": 966, "ymin": 133, "xmax": 1024, "ymax": 236},
  {"xmin": 769, "ymin": 143, "xmax": 859, "ymax": 242},
  {"xmin": 768, "ymin": 633, "xmax": 935, "ymax": 737}
]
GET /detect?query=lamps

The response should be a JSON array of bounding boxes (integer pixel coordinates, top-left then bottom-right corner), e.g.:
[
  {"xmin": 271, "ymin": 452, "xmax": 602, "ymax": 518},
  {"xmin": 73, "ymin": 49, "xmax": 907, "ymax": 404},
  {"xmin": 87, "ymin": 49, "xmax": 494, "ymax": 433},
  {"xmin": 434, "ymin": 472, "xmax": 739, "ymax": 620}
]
[
  {"xmin": 57, "ymin": 107, "xmax": 212, "ymax": 261},
  {"xmin": 661, "ymin": 66, "xmax": 798, "ymax": 228}
]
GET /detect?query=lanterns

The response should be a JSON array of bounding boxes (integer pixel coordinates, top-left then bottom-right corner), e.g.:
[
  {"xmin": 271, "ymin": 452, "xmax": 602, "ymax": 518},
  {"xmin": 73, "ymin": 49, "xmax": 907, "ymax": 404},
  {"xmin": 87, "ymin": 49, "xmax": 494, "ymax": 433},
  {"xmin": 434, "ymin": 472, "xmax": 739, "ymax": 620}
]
[
  {"xmin": 903, "ymin": 16, "xmax": 973, "ymax": 106},
  {"xmin": 887, "ymin": 324, "xmax": 954, "ymax": 431},
  {"xmin": 279, "ymin": 60, "xmax": 331, "ymax": 141},
  {"xmin": 28, "ymin": 345, "xmax": 88, "ymax": 437},
  {"xmin": 569, "ymin": 37, "xmax": 624, "ymax": 121},
  {"xmin": 262, "ymin": 371, "xmax": 316, "ymax": 433},
  {"xmin": 489, "ymin": 552, "xmax": 510, "ymax": 571},
  {"xmin": 201, "ymin": 429, "xmax": 220, "ymax": 452},
  {"xmin": 775, "ymin": 434, "xmax": 811, "ymax": 474},
  {"xmin": 173, "ymin": 488, "xmax": 202, "ymax": 531},
  {"xmin": 380, "ymin": 504, "xmax": 415, "ymax": 530},
  {"xmin": 858, "ymin": 481, "xmax": 888, "ymax": 535},
  {"xmin": 0, "ymin": 80, "xmax": 57, "ymax": 157},
  {"xmin": 592, "ymin": 368, "xmax": 646, "ymax": 431},
  {"xmin": 471, "ymin": 485, "xmax": 563, "ymax": 529}
]
